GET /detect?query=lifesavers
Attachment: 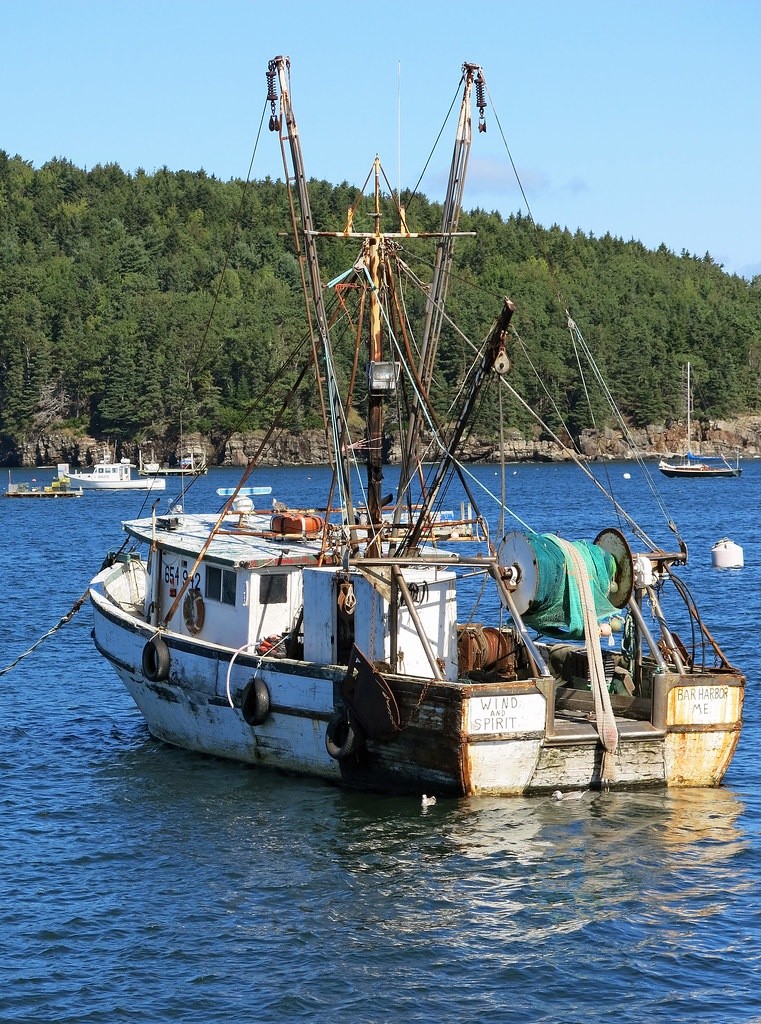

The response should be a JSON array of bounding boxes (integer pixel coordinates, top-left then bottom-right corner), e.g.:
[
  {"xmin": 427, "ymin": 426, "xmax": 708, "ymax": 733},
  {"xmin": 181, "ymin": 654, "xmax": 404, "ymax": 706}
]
[
  {"xmin": 325, "ymin": 713, "xmax": 365, "ymax": 761},
  {"xmin": 241, "ymin": 678, "xmax": 270, "ymax": 727},
  {"xmin": 270, "ymin": 512, "xmax": 325, "ymax": 534},
  {"xmin": 142, "ymin": 638, "xmax": 169, "ymax": 682},
  {"xmin": 182, "ymin": 588, "xmax": 204, "ymax": 633}
]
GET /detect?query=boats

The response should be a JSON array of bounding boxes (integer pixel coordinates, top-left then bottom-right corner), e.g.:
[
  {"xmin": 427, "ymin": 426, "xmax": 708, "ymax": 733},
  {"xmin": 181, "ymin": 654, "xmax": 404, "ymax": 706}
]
[
  {"xmin": 80, "ymin": 50, "xmax": 748, "ymax": 808},
  {"xmin": 63, "ymin": 439, "xmax": 166, "ymax": 492},
  {"xmin": 5, "ymin": 468, "xmax": 85, "ymax": 498}
]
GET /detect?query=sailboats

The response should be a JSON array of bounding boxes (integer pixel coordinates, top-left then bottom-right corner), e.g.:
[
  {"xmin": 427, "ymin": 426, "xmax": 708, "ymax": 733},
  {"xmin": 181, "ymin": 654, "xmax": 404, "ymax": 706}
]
[{"xmin": 658, "ymin": 360, "xmax": 745, "ymax": 479}]
